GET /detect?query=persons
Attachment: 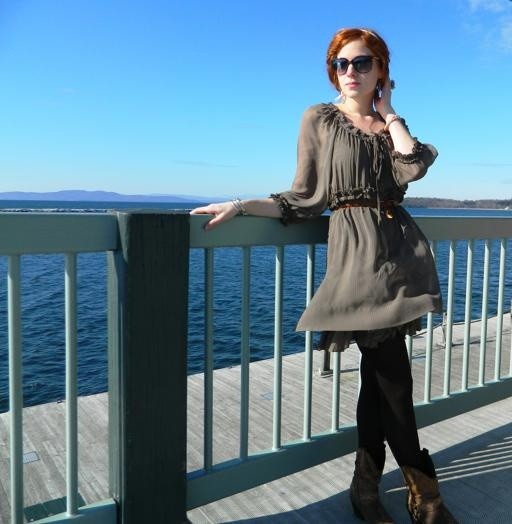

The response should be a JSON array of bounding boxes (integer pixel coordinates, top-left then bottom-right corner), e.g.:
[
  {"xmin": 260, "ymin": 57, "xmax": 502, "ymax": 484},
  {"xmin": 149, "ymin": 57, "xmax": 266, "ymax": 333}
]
[{"xmin": 190, "ymin": 25, "xmax": 456, "ymax": 524}]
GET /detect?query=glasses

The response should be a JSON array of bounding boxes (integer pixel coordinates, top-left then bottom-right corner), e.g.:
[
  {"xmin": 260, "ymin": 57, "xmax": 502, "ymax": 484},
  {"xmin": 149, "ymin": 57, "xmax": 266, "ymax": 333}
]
[{"xmin": 330, "ymin": 56, "xmax": 376, "ymax": 75}]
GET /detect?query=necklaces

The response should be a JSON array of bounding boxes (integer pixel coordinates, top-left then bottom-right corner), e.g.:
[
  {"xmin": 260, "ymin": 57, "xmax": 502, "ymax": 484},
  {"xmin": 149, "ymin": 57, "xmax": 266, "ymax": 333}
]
[{"xmin": 340, "ymin": 110, "xmax": 377, "ymax": 134}]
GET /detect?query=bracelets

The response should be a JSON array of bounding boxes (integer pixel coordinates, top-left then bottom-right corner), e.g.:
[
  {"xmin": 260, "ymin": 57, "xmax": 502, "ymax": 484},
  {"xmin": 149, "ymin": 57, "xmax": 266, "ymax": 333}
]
[
  {"xmin": 383, "ymin": 114, "xmax": 401, "ymax": 132},
  {"xmin": 229, "ymin": 198, "xmax": 248, "ymax": 217}
]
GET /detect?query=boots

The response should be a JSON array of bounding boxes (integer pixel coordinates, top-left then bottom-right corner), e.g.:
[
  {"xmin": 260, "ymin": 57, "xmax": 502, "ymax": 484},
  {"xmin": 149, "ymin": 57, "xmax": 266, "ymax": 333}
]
[
  {"xmin": 349, "ymin": 442, "xmax": 397, "ymax": 524},
  {"xmin": 397, "ymin": 448, "xmax": 459, "ymax": 523}
]
